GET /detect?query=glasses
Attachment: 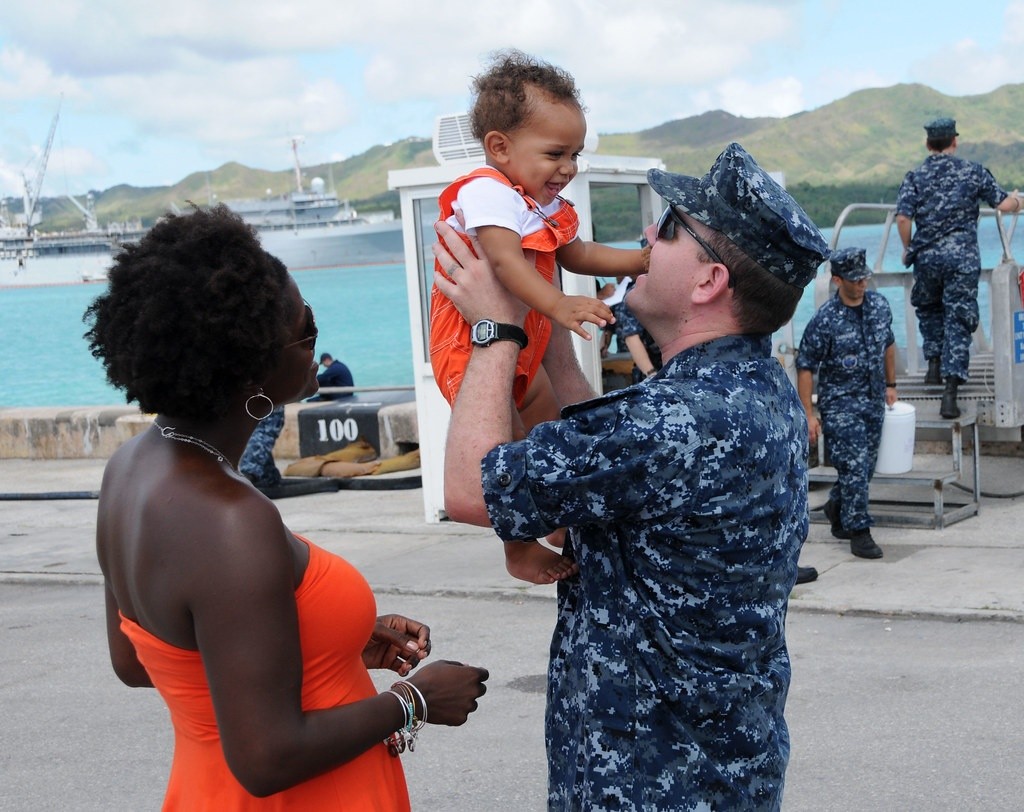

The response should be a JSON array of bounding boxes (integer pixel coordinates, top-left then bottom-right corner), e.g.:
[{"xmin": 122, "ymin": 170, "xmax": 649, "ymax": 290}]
[
  {"xmin": 655, "ymin": 203, "xmax": 735, "ymax": 288},
  {"xmin": 271, "ymin": 298, "xmax": 320, "ymax": 350},
  {"xmin": 845, "ymin": 275, "xmax": 872, "ymax": 285}
]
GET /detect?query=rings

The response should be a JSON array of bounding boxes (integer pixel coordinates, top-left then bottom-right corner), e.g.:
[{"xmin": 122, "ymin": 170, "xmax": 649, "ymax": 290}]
[{"xmin": 447, "ymin": 264, "xmax": 459, "ymax": 276}]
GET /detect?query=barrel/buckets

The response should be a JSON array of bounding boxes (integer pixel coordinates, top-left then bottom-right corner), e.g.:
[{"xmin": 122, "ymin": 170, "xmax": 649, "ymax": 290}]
[{"xmin": 873, "ymin": 402, "xmax": 917, "ymax": 474}]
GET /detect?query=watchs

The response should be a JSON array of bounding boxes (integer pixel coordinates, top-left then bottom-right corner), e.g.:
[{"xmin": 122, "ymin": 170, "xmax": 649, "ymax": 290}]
[{"xmin": 470, "ymin": 319, "xmax": 529, "ymax": 350}]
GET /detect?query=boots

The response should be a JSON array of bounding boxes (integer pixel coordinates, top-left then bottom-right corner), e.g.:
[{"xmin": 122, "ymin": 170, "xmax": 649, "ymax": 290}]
[
  {"xmin": 924, "ymin": 357, "xmax": 942, "ymax": 384},
  {"xmin": 940, "ymin": 376, "xmax": 961, "ymax": 418}
]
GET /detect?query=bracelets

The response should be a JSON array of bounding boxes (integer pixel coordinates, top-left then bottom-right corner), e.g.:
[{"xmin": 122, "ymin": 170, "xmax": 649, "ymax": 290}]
[
  {"xmin": 1015, "ymin": 198, "xmax": 1019, "ymax": 209},
  {"xmin": 646, "ymin": 369, "xmax": 655, "ymax": 376},
  {"xmin": 886, "ymin": 383, "xmax": 896, "ymax": 387},
  {"xmin": 381, "ymin": 681, "xmax": 427, "ymax": 757}
]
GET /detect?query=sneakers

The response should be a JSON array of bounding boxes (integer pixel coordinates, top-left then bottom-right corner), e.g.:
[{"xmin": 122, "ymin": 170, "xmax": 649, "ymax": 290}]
[
  {"xmin": 824, "ymin": 500, "xmax": 850, "ymax": 539},
  {"xmin": 849, "ymin": 528, "xmax": 882, "ymax": 557},
  {"xmin": 796, "ymin": 567, "xmax": 817, "ymax": 585}
]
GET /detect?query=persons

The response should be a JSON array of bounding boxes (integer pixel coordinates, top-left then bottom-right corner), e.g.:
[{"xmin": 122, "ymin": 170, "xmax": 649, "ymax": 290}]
[
  {"xmin": 306, "ymin": 352, "xmax": 355, "ymax": 402},
  {"xmin": 237, "ymin": 404, "xmax": 285, "ymax": 485},
  {"xmin": 428, "ymin": 50, "xmax": 643, "ymax": 584},
  {"xmin": 591, "ymin": 226, "xmax": 663, "ymax": 385},
  {"xmin": 433, "ymin": 143, "xmax": 833, "ymax": 812},
  {"xmin": 795, "ymin": 248, "xmax": 897, "ymax": 559},
  {"xmin": 894, "ymin": 118, "xmax": 1024, "ymax": 419},
  {"xmin": 84, "ymin": 204, "xmax": 489, "ymax": 812}
]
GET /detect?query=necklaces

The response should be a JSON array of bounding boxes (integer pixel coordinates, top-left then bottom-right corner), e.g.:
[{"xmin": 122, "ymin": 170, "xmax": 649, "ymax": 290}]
[{"xmin": 154, "ymin": 422, "xmax": 233, "ymax": 472}]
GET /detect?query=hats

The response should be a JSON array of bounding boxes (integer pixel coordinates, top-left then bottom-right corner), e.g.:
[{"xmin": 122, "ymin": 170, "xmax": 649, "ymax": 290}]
[
  {"xmin": 318, "ymin": 353, "xmax": 329, "ymax": 364},
  {"xmin": 830, "ymin": 246, "xmax": 873, "ymax": 281},
  {"xmin": 650, "ymin": 144, "xmax": 831, "ymax": 288},
  {"xmin": 924, "ymin": 118, "xmax": 959, "ymax": 137}
]
